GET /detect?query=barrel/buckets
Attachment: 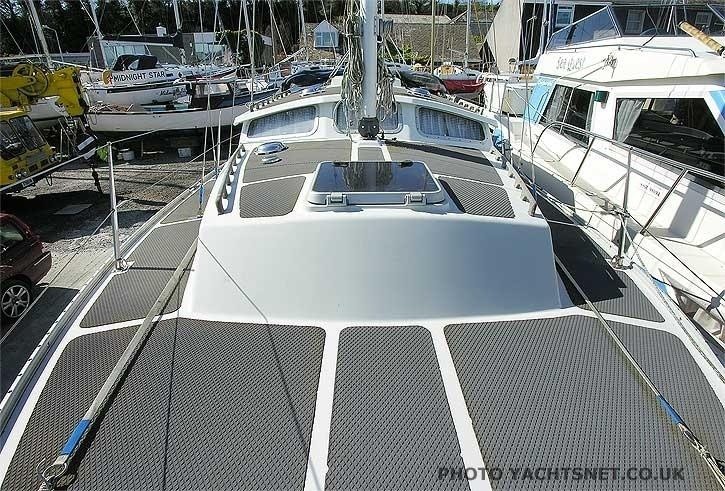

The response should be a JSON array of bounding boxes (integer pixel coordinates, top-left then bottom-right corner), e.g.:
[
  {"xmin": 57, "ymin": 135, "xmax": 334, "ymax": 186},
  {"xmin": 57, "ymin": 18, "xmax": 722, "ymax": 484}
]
[{"xmin": 123, "ymin": 151, "xmax": 134, "ymax": 161}]
